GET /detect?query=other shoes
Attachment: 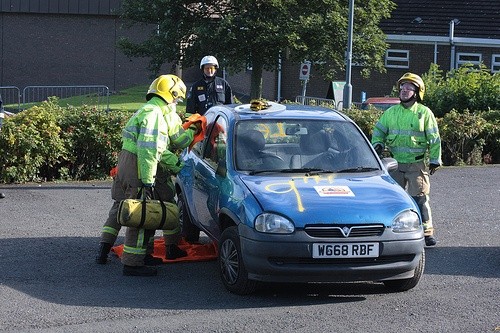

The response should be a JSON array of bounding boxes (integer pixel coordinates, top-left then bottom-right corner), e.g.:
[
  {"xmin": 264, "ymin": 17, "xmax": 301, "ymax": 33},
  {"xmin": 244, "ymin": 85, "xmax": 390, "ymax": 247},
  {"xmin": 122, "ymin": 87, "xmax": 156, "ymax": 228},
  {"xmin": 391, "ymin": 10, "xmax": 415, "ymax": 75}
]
[
  {"xmin": 143, "ymin": 255, "xmax": 163, "ymax": 265},
  {"xmin": 95, "ymin": 242, "xmax": 112, "ymax": 264},
  {"xmin": 124, "ymin": 264, "xmax": 157, "ymax": 277},
  {"xmin": 426, "ymin": 235, "xmax": 436, "ymax": 246},
  {"xmin": 165, "ymin": 245, "xmax": 187, "ymax": 260}
]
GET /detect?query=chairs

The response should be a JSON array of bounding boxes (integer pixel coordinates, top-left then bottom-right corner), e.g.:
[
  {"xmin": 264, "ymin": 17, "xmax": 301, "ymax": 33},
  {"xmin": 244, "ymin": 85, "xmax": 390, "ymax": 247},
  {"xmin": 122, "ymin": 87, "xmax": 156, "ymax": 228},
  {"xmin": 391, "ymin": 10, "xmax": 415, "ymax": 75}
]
[
  {"xmin": 236, "ymin": 130, "xmax": 264, "ymax": 169},
  {"xmin": 291, "ymin": 130, "xmax": 335, "ymax": 169}
]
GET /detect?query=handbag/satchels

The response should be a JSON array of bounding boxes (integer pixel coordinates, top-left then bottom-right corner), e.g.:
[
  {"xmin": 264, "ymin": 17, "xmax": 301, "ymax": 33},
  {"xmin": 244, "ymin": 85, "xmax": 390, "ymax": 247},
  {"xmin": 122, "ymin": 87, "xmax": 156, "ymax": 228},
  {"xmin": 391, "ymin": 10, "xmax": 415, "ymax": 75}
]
[{"xmin": 116, "ymin": 184, "xmax": 179, "ymax": 230}]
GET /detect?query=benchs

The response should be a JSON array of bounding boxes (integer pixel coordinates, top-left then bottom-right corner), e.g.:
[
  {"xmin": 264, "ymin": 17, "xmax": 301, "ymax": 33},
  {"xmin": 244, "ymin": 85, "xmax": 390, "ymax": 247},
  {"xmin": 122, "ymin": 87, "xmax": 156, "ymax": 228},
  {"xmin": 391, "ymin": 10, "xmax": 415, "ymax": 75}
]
[{"xmin": 214, "ymin": 144, "xmax": 300, "ymax": 166}]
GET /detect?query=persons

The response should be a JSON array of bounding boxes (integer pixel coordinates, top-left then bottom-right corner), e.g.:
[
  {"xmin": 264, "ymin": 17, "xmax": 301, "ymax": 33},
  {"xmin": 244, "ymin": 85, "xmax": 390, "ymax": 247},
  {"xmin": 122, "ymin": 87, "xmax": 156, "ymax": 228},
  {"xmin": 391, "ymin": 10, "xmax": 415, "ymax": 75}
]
[
  {"xmin": 186, "ymin": 55, "xmax": 233, "ymax": 115},
  {"xmin": 371, "ymin": 73, "xmax": 443, "ymax": 246},
  {"xmin": 94, "ymin": 73, "xmax": 196, "ymax": 277},
  {"xmin": 177, "ymin": 138, "xmax": 230, "ymax": 225},
  {"xmin": 0, "ymin": 95, "xmax": 6, "ymax": 198}
]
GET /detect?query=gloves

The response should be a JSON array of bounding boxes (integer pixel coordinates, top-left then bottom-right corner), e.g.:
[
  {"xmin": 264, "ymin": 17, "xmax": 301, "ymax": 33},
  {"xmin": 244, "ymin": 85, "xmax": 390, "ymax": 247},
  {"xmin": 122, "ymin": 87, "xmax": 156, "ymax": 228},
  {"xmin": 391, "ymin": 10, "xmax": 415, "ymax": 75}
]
[
  {"xmin": 429, "ymin": 163, "xmax": 440, "ymax": 175},
  {"xmin": 374, "ymin": 144, "xmax": 390, "ymax": 159}
]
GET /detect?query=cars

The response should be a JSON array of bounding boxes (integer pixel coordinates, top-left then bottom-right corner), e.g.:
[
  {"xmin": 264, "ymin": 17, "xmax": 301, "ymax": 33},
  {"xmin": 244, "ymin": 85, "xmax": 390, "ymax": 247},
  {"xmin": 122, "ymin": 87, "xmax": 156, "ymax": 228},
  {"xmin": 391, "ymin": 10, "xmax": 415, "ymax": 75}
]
[{"xmin": 173, "ymin": 99, "xmax": 427, "ymax": 295}]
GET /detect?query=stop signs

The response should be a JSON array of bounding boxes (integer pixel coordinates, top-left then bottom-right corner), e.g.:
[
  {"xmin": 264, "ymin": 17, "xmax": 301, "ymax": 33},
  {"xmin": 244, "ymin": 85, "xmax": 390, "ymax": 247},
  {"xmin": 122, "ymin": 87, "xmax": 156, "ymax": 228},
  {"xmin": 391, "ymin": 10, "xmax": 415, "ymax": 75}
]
[{"xmin": 299, "ymin": 63, "xmax": 311, "ymax": 80}]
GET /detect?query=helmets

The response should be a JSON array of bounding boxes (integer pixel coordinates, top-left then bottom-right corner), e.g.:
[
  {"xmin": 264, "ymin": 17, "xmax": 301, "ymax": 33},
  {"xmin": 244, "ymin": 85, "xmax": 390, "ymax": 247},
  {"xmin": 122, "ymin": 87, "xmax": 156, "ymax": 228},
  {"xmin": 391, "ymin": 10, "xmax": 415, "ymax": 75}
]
[
  {"xmin": 200, "ymin": 55, "xmax": 219, "ymax": 70},
  {"xmin": 146, "ymin": 74, "xmax": 187, "ymax": 104},
  {"xmin": 397, "ymin": 73, "xmax": 425, "ymax": 101}
]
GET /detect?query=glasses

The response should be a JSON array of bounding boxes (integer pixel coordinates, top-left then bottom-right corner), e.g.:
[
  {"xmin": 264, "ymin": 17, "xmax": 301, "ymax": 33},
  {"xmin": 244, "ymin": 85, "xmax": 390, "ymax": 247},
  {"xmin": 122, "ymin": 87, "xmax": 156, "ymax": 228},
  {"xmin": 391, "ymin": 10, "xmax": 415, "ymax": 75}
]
[{"xmin": 399, "ymin": 85, "xmax": 416, "ymax": 91}]
